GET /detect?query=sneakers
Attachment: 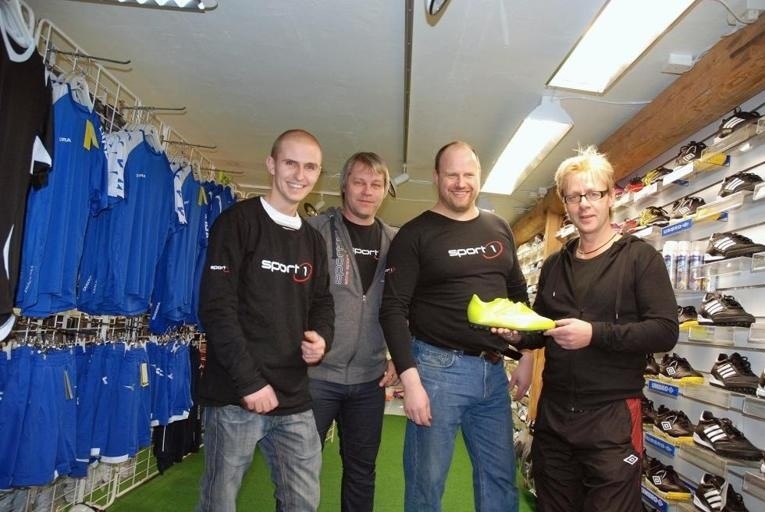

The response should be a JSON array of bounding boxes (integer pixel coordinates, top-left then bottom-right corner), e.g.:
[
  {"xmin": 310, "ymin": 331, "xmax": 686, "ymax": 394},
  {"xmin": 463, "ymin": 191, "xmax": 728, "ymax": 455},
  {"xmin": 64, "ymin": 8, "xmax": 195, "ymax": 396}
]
[
  {"xmin": 697, "ymin": 292, "xmax": 755, "ymax": 327},
  {"xmin": 611, "ymin": 141, "xmax": 706, "ymax": 234},
  {"xmin": 641, "ymin": 304, "xmax": 706, "ymax": 501},
  {"xmin": 716, "ymin": 171, "xmax": 765, "ymax": 197},
  {"xmin": 713, "ymin": 106, "xmax": 765, "ymax": 142},
  {"xmin": 708, "ymin": 352, "xmax": 761, "ymax": 394},
  {"xmin": 466, "ymin": 293, "xmax": 557, "ymax": 334},
  {"xmin": 704, "ymin": 232, "xmax": 765, "ymax": 262},
  {"xmin": 693, "ymin": 410, "xmax": 763, "ymax": 461},
  {"xmin": 693, "ymin": 472, "xmax": 749, "ymax": 511}
]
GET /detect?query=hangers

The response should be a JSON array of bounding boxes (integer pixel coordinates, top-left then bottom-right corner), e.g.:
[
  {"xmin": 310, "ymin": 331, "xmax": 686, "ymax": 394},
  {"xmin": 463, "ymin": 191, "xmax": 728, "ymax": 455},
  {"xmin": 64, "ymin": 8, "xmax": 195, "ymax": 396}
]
[{"xmin": 42, "ymin": 43, "xmax": 244, "ymax": 202}]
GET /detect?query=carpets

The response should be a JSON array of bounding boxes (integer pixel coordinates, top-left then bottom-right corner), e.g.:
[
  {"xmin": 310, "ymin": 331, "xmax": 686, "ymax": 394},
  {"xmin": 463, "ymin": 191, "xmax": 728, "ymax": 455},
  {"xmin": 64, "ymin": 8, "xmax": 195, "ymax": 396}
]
[{"xmin": 36, "ymin": 410, "xmax": 541, "ymax": 512}]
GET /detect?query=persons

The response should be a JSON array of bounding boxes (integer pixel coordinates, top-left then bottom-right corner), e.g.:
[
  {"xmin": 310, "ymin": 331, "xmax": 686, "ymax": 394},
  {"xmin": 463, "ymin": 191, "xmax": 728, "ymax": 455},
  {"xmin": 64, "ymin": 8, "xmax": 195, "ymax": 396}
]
[
  {"xmin": 489, "ymin": 145, "xmax": 681, "ymax": 512},
  {"xmin": 274, "ymin": 150, "xmax": 398, "ymax": 512},
  {"xmin": 195, "ymin": 128, "xmax": 336, "ymax": 511},
  {"xmin": 380, "ymin": 140, "xmax": 536, "ymax": 512}
]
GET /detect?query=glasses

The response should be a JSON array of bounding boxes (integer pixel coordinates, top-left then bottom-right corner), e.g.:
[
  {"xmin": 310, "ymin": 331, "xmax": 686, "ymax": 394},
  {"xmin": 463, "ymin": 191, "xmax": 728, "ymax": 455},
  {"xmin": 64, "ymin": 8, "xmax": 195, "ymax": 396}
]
[{"xmin": 561, "ymin": 190, "xmax": 610, "ymax": 203}]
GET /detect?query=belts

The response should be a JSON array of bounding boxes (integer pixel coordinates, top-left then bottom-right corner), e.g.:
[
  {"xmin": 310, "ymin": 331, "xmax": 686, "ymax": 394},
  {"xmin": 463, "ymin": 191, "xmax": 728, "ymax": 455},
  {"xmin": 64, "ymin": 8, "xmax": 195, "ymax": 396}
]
[{"xmin": 448, "ymin": 349, "xmax": 523, "ymax": 365}]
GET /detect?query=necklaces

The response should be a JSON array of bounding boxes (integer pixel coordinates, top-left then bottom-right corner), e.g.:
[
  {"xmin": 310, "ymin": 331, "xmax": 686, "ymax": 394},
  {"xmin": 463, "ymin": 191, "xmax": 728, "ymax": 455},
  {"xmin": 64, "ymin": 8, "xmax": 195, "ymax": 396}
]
[{"xmin": 577, "ymin": 232, "xmax": 619, "ymax": 255}]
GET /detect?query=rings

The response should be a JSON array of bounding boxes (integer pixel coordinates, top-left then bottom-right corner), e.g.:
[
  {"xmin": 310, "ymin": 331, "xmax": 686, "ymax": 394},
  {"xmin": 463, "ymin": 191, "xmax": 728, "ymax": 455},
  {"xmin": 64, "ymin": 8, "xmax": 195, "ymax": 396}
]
[
  {"xmin": 506, "ymin": 333, "xmax": 513, "ymax": 338},
  {"xmin": 385, "ymin": 371, "xmax": 392, "ymax": 378}
]
